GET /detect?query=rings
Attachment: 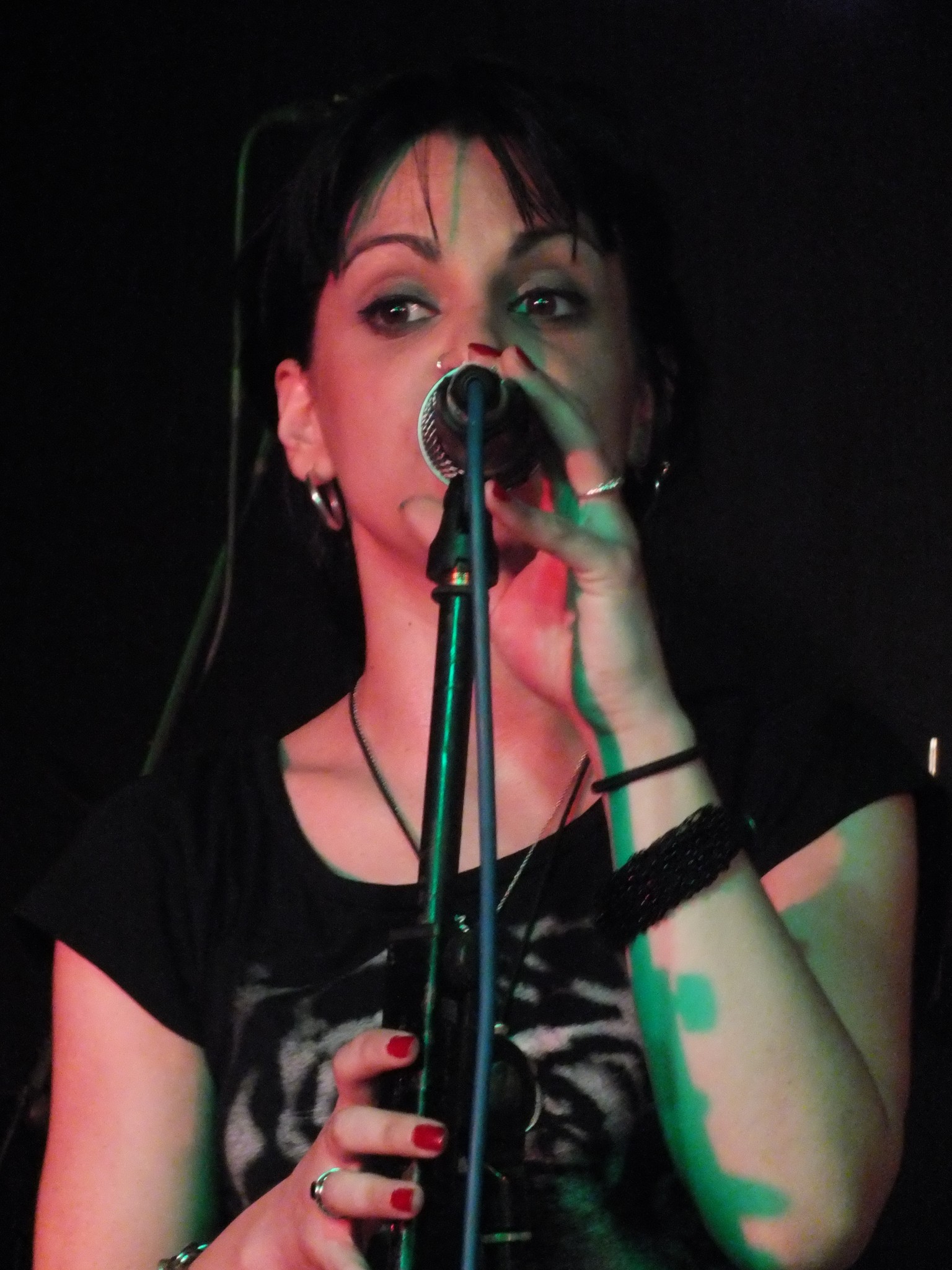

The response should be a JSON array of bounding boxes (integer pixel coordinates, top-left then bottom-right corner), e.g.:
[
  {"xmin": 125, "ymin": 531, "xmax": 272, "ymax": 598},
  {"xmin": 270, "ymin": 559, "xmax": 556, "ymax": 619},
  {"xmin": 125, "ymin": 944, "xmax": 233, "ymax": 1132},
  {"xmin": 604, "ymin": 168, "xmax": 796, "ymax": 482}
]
[
  {"xmin": 310, "ymin": 1168, "xmax": 350, "ymax": 1219},
  {"xmin": 574, "ymin": 476, "xmax": 621, "ymax": 503}
]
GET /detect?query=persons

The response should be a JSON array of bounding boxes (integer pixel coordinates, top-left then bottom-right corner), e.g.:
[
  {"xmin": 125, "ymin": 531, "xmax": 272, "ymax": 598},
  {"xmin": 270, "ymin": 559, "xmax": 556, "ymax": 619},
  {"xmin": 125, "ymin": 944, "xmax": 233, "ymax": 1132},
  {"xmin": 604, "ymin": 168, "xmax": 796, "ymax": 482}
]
[{"xmin": 31, "ymin": 102, "xmax": 926, "ymax": 1270}]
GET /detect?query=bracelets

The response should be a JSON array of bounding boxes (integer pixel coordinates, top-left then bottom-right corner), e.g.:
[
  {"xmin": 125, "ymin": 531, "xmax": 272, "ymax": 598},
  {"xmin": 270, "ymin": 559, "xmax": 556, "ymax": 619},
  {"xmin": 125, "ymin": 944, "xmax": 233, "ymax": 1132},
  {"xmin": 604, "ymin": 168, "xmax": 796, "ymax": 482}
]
[
  {"xmin": 590, "ymin": 740, "xmax": 707, "ymax": 792},
  {"xmin": 156, "ymin": 1242, "xmax": 208, "ymax": 1270},
  {"xmin": 599, "ymin": 799, "xmax": 748, "ymax": 952}
]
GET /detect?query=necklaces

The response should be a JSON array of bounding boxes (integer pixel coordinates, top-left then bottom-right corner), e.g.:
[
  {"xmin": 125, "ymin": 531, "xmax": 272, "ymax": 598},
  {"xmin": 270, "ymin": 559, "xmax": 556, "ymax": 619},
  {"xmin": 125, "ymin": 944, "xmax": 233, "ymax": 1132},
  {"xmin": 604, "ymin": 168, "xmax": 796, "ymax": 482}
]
[{"xmin": 351, "ymin": 682, "xmax": 593, "ymax": 1136}]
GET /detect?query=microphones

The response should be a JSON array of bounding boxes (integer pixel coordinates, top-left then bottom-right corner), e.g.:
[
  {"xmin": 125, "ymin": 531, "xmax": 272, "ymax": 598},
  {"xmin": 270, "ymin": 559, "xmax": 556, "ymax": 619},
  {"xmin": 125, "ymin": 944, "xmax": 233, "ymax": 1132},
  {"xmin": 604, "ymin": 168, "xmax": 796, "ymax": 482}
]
[{"xmin": 418, "ymin": 360, "xmax": 549, "ymax": 494}]
